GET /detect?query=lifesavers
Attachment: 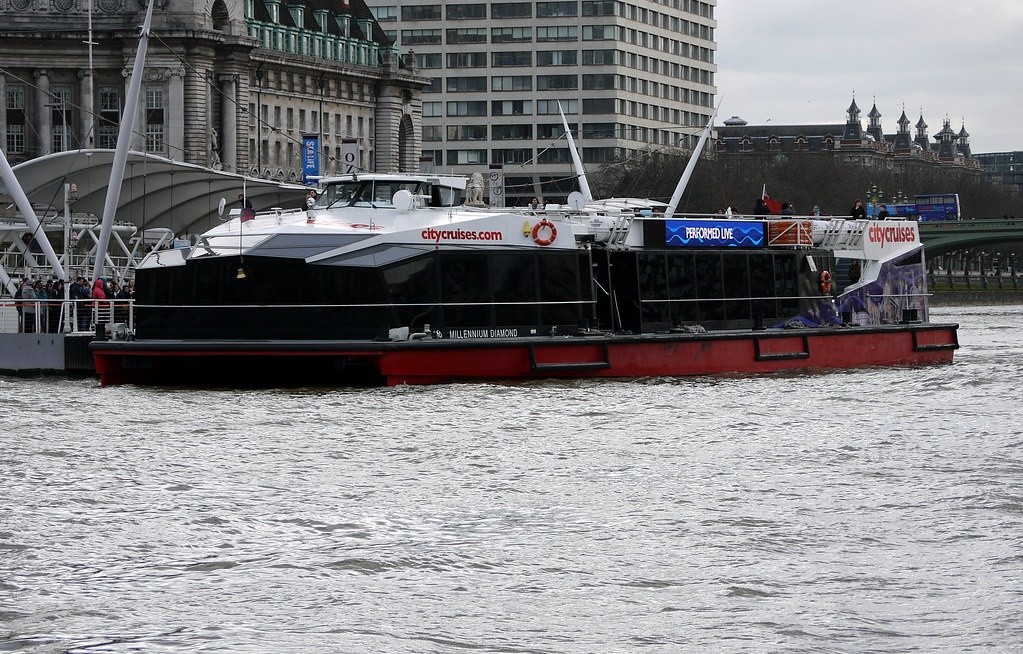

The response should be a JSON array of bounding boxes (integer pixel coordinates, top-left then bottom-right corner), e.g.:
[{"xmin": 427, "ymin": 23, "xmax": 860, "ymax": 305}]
[
  {"xmin": 820, "ymin": 270, "xmax": 832, "ymax": 295},
  {"xmin": 532, "ymin": 220, "xmax": 558, "ymax": 246}
]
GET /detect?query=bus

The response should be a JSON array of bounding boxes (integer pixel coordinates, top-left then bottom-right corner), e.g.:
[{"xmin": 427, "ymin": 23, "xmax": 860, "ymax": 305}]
[{"xmin": 865, "ymin": 193, "xmax": 961, "ymax": 221}]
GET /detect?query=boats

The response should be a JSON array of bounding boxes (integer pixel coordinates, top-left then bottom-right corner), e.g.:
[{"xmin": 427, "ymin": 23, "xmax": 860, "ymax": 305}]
[{"xmin": 89, "ymin": 169, "xmax": 962, "ymax": 390}]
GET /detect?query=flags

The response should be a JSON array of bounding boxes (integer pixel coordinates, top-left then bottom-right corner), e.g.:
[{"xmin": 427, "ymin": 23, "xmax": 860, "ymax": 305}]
[{"xmin": 763, "ymin": 190, "xmax": 783, "ymax": 215}]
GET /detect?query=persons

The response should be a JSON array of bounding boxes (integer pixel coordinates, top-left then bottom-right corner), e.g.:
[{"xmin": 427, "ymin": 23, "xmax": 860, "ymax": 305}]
[
  {"xmin": 847, "ymin": 258, "xmax": 861, "ymax": 284},
  {"xmin": 715, "ymin": 207, "xmax": 745, "ymax": 220},
  {"xmin": 306, "ymin": 189, "xmax": 320, "ymax": 210},
  {"xmin": 649, "ymin": 207, "xmax": 666, "ymax": 218},
  {"xmin": 239, "ymin": 192, "xmax": 252, "ymax": 211},
  {"xmin": 529, "ymin": 198, "xmax": 542, "ymax": 217},
  {"xmin": 753, "ymin": 198, "xmax": 770, "ymax": 222},
  {"xmin": 875, "ymin": 202, "xmax": 890, "ymax": 220},
  {"xmin": 13, "ymin": 275, "xmax": 135, "ymax": 334},
  {"xmin": 633, "ymin": 207, "xmax": 645, "ymax": 218},
  {"xmin": 850, "ymin": 199, "xmax": 868, "ymax": 220},
  {"xmin": 780, "ymin": 202, "xmax": 796, "ymax": 220},
  {"xmin": 807, "ymin": 205, "xmax": 832, "ymax": 221}
]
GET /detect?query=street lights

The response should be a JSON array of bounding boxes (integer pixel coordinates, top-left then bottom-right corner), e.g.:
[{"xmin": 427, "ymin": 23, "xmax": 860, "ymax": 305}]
[{"xmin": 866, "ymin": 182, "xmax": 884, "ymax": 220}]
